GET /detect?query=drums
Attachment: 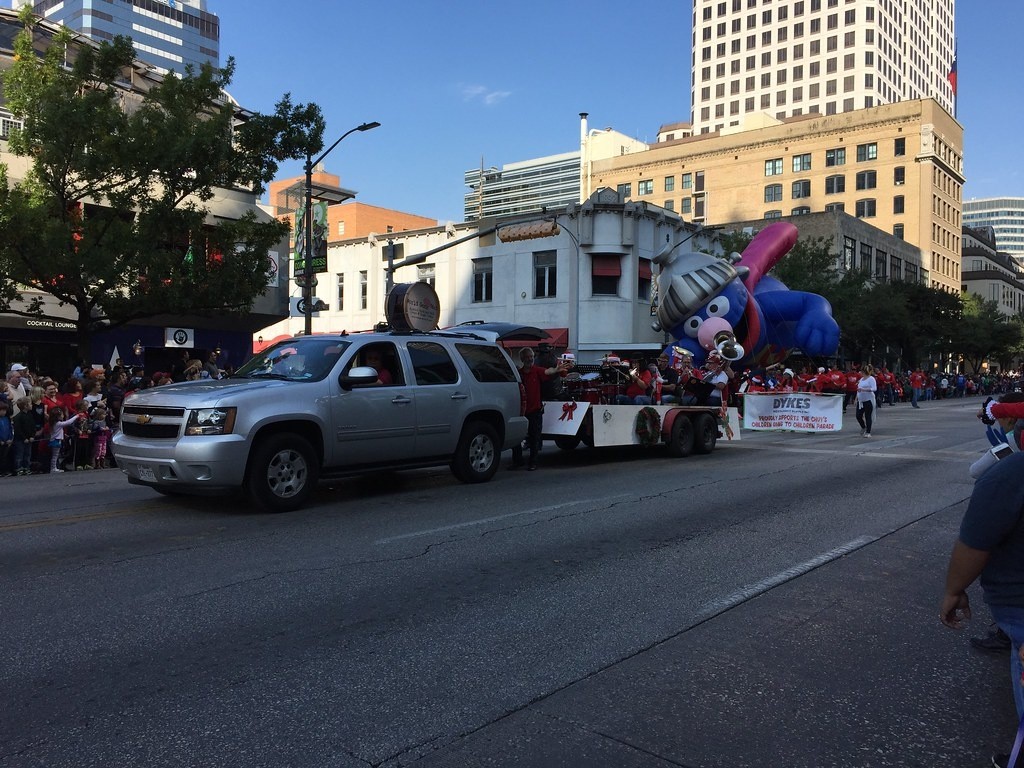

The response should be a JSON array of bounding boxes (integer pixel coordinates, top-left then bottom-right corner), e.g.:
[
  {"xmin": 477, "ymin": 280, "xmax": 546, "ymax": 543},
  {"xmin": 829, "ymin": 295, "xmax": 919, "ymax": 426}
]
[{"xmin": 384, "ymin": 281, "xmax": 441, "ymax": 332}]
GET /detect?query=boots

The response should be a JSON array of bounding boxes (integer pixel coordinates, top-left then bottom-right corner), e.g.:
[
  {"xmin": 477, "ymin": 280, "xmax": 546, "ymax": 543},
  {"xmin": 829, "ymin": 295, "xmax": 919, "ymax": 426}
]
[
  {"xmin": 94, "ymin": 459, "xmax": 102, "ymax": 469},
  {"xmin": 100, "ymin": 458, "xmax": 109, "ymax": 470}
]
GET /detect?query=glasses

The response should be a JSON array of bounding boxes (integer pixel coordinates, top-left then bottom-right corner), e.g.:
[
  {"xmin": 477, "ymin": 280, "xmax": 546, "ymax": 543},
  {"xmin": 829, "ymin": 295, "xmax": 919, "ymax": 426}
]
[{"xmin": 683, "ymin": 362, "xmax": 690, "ymax": 363}]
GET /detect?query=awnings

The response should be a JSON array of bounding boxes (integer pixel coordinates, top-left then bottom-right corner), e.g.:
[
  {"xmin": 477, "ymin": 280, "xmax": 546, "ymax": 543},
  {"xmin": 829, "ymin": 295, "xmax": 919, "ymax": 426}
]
[{"xmin": 0, "ymin": 293, "xmax": 77, "ymax": 331}]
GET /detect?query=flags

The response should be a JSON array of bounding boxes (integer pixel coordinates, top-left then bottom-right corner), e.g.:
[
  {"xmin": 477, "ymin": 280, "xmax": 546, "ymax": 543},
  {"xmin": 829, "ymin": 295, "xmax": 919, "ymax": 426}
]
[{"xmin": 949, "ymin": 43, "xmax": 957, "ymax": 95}]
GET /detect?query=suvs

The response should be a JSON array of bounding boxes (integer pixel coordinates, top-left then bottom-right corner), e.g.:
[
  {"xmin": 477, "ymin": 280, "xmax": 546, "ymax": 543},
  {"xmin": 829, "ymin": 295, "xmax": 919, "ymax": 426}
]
[{"xmin": 110, "ymin": 319, "xmax": 554, "ymax": 515}]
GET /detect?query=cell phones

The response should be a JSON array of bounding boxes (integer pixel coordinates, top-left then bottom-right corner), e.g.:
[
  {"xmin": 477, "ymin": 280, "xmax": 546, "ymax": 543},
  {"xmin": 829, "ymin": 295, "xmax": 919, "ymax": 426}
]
[{"xmin": 990, "ymin": 442, "xmax": 1015, "ymax": 460}]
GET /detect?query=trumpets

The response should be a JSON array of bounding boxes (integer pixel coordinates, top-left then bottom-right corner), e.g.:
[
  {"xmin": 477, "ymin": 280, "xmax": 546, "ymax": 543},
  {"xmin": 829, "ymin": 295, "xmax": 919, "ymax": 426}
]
[{"xmin": 687, "ymin": 367, "xmax": 697, "ymax": 384}]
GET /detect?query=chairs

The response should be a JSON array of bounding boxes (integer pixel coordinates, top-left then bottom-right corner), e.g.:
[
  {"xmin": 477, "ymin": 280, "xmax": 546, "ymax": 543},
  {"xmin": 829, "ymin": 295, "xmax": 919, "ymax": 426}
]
[
  {"xmin": 321, "ymin": 352, "xmax": 338, "ymax": 372},
  {"xmin": 383, "ymin": 355, "xmax": 399, "ymax": 385}
]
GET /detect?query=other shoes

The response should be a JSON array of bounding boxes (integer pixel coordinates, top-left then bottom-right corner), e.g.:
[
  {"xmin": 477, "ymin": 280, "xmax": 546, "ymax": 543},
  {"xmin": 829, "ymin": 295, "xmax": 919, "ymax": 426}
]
[
  {"xmin": 76, "ymin": 466, "xmax": 83, "ymax": 472},
  {"xmin": 84, "ymin": 465, "xmax": 94, "ymax": 471},
  {"xmin": 49, "ymin": 468, "xmax": 65, "ymax": 474},
  {"xmin": 861, "ymin": 426, "xmax": 868, "ymax": 436},
  {"xmin": 529, "ymin": 462, "xmax": 538, "ymax": 471},
  {"xmin": 864, "ymin": 432, "xmax": 872, "ymax": 438},
  {"xmin": 890, "ymin": 403, "xmax": 896, "ymax": 406},
  {"xmin": 507, "ymin": 459, "xmax": 525, "ymax": 471},
  {"xmin": 914, "ymin": 405, "xmax": 920, "ymax": 409},
  {"xmin": 992, "ymin": 754, "xmax": 1021, "ymax": 768},
  {"xmin": 17, "ymin": 468, "xmax": 32, "ymax": 477}
]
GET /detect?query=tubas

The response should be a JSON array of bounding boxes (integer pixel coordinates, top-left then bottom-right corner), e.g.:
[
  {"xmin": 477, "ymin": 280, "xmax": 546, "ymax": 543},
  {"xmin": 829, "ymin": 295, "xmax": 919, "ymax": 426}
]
[
  {"xmin": 671, "ymin": 344, "xmax": 695, "ymax": 372},
  {"xmin": 713, "ymin": 329, "xmax": 744, "ymax": 372}
]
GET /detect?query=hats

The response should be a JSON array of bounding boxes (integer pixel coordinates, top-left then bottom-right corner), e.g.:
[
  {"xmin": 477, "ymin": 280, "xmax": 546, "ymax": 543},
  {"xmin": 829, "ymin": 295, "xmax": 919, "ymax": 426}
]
[
  {"xmin": 751, "ymin": 376, "xmax": 766, "ymax": 386},
  {"xmin": 648, "ymin": 359, "xmax": 659, "ymax": 367},
  {"xmin": 90, "ymin": 368, "xmax": 106, "ymax": 379},
  {"xmin": 630, "ymin": 352, "xmax": 644, "ymax": 362},
  {"xmin": 782, "ymin": 368, "xmax": 794, "ymax": 378},
  {"xmin": 11, "ymin": 364, "xmax": 27, "ymax": 372},
  {"xmin": 557, "ymin": 352, "xmax": 575, "ymax": 359},
  {"xmin": 608, "ymin": 354, "xmax": 621, "ymax": 363},
  {"xmin": 621, "ymin": 360, "xmax": 630, "ymax": 366},
  {"xmin": 705, "ymin": 351, "xmax": 721, "ymax": 363},
  {"xmin": 767, "ymin": 378, "xmax": 778, "ymax": 388}
]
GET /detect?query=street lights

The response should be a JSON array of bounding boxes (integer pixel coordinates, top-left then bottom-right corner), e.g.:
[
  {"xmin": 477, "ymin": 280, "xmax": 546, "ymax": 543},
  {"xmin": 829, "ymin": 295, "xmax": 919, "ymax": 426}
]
[{"xmin": 303, "ymin": 120, "xmax": 382, "ymax": 336}]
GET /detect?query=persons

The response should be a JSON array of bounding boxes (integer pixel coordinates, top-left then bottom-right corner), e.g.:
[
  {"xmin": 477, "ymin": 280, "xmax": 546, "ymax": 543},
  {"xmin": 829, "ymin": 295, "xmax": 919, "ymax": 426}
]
[
  {"xmin": 0, "ymin": 348, "xmax": 231, "ymax": 477},
  {"xmin": 855, "ymin": 364, "xmax": 877, "ymax": 438},
  {"xmin": 506, "ymin": 347, "xmax": 570, "ymax": 471},
  {"xmin": 341, "ymin": 350, "xmax": 396, "ymax": 387},
  {"xmin": 941, "ymin": 392, "xmax": 1024, "ymax": 768},
  {"xmin": 535, "ymin": 350, "xmax": 1024, "ymax": 417}
]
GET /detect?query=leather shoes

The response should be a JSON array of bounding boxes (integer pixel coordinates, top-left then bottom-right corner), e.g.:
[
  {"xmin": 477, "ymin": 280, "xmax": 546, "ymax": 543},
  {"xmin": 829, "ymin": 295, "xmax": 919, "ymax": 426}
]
[{"xmin": 969, "ymin": 628, "xmax": 1012, "ymax": 655}]
